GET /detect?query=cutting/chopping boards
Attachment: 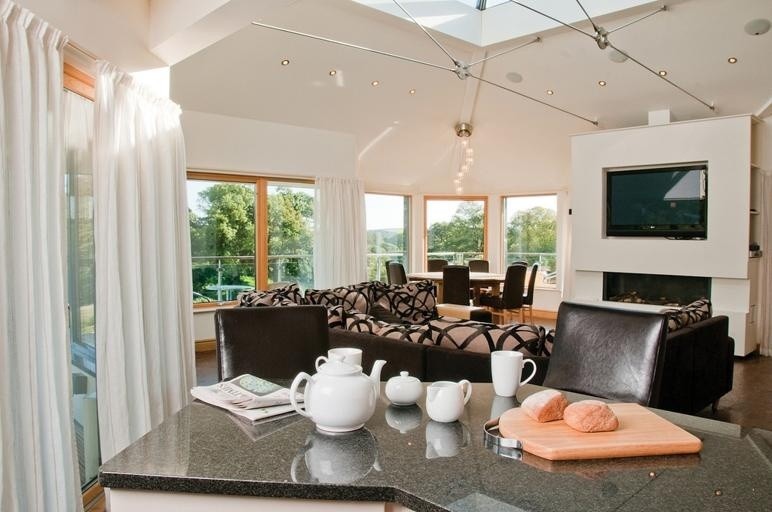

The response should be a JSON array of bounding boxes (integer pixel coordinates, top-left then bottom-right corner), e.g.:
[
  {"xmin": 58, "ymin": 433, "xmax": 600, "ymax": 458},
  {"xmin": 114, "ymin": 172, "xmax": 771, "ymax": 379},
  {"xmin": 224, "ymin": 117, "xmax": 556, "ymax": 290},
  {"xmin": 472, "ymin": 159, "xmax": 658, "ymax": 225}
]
[
  {"xmin": 485, "ymin": 403, "xmax": 702, "ymax": 462},
  {"xmin": 485, "ymin": 442, "xmax": 700, "ymax": 474}
]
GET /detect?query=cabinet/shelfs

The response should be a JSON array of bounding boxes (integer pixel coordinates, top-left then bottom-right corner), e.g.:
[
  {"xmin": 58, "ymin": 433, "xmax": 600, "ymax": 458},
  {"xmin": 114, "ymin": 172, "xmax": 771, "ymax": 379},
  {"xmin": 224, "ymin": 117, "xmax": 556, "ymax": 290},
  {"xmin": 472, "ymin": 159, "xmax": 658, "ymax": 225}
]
[{"xmin": 747, "ymin": 118, "xmax": 771, "ymax": 359}]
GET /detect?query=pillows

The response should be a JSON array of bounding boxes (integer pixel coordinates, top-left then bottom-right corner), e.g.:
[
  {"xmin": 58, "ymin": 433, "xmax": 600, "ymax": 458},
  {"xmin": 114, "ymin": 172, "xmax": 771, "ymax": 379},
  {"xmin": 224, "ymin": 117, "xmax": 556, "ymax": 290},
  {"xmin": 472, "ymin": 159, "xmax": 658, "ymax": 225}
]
[
  {"xmin": 426, "ymin": 319, "xmax": 540, "ymax": 363},
  {"xmin": 345, "ymin": 309, "xmax": 423, "ymax": 344},
  {"xmin": 681, "ymin": 300, "xmax": 711, "ymax": 327}
]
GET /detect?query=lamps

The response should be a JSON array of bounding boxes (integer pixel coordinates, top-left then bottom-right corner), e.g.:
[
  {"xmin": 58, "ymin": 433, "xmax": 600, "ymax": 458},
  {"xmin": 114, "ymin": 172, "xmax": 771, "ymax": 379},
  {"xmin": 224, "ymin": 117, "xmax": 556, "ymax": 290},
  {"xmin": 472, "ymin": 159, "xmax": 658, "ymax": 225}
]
[{"xmin": 453, "ymin": 123, "xmax": 478, "ymax": 194}]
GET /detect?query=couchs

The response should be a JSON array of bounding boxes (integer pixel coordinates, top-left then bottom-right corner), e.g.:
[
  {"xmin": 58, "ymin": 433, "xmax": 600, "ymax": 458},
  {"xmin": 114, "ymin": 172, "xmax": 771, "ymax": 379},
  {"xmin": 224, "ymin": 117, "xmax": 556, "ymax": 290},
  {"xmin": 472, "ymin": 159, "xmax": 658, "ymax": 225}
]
[
  {"xmin": 654, "ymin": 313, "xmax": 746, "ymax": 419},
  {"xmin": 208, "ymin": 283, "xmax": 553, "ymax": 387}
]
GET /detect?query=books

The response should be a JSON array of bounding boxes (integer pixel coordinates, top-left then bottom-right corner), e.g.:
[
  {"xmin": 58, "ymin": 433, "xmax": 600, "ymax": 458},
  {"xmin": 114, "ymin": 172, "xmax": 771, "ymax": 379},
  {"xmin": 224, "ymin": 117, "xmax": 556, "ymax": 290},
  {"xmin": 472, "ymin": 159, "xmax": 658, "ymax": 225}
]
[{"xmin": 190, "ymin": 372, "xmax": 307, "ymax": 421}]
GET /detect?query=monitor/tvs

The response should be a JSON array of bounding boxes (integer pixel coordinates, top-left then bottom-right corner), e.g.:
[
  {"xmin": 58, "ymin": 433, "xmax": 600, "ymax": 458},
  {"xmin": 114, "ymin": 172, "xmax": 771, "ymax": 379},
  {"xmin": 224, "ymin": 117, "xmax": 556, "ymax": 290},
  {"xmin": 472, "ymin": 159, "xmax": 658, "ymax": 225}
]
[{"xmin": 606, "ymin": 165, "xmax": 708, "ymax": 237}]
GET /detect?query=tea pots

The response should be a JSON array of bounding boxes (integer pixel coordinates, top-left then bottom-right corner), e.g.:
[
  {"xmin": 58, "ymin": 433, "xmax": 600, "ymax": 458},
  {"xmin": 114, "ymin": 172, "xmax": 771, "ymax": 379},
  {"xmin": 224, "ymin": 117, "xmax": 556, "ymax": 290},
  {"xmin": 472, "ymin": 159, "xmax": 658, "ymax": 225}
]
[
  {"xmin": 383, "ymin": 371, "xmax": 423, "ymax": 408},
  {"xmin": 383, "ymin": 403, "xmax": 420, "ymax": 436},
  {"xmin": 288, "ymin": 353, "xmax": 385, "ymax": 434},
  {"xmin": 428, "ymin": 380, "xmax": 474, "ymax": 421},
  {"xmin": 424, "ymin": 422, "xmax": 473, "ymax": 457},
  {"xmin": 290, "ymin": 428, "xmax": 383, "ymax": 485}
]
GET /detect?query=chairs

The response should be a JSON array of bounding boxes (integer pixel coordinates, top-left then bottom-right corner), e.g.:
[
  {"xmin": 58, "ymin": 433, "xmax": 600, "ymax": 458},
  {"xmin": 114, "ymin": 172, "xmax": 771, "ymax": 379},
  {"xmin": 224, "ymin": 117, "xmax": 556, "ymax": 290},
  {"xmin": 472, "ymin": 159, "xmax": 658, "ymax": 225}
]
[
  {"xmin": 384, "ymin": 258, "xmax": 543, "ymax": 321},
  {"xmin": 210, "ymin": 303, "xmax": 334, "ymax": 384},
  {"xmin": 537, "ymin": 301, "xmax": 670, "ymax": 409}
]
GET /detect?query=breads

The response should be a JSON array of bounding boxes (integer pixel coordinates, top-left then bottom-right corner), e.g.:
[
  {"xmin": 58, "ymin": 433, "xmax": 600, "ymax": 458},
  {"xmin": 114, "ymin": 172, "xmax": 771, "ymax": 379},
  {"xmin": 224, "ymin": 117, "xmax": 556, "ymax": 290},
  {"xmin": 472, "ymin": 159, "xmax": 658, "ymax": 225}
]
[
  {"xmin": 520, "ymin": 388, "xmax": 567, "ymax": 424},
  {"xmin": 561, "ymin": 399, "xmax": 619, "ymax": 433}
]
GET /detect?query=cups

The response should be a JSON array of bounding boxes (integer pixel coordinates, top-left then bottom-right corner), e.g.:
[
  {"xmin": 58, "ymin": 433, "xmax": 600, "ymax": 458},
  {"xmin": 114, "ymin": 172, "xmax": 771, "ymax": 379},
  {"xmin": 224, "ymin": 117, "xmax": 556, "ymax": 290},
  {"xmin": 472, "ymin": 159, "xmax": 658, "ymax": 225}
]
[
  {"xmin": 315, "ymin": 347, "xmax": 363, "ymax": 374},
  {"xmin": 489, "ymin": 396, "xmax": 520, "ymax": 423},
  {"xmin": 490, "ymin": 348, "xmax": 537, "ymax": 398}
]
